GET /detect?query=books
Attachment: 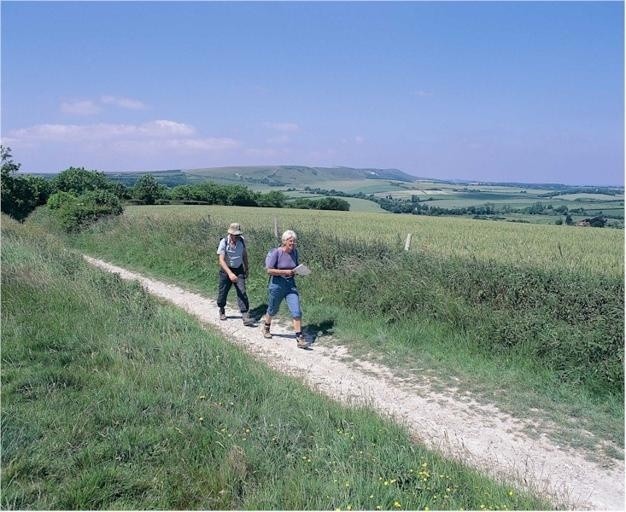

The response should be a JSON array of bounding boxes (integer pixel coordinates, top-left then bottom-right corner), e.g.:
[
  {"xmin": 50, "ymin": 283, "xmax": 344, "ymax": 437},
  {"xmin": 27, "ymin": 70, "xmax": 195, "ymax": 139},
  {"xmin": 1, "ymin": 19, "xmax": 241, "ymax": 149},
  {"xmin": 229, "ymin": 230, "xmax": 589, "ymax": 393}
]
[{"xmin": 291, "ymin": 262, "xmax": 312, "ymax": 278}]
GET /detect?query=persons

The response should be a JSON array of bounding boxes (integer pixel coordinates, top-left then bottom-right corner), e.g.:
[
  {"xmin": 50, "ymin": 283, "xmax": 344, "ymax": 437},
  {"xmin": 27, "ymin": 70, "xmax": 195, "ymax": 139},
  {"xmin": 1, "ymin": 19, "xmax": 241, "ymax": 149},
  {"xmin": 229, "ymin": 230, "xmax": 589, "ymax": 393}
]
[
  {"xmin": 216, "ymin": 221, "xmax": 256, "ymax": 326},
  {"xmin": 264, "ymin": 229, "xmax": 310, "ymax": 349}
]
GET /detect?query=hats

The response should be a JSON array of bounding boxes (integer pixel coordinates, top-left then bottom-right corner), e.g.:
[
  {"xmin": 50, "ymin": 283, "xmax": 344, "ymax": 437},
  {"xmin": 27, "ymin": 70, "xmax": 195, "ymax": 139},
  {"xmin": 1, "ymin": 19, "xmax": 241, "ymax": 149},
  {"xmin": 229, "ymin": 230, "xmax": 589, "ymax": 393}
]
[{"xmin": 228, "ymin": 223, "xmax": 243, "ymax": 235}]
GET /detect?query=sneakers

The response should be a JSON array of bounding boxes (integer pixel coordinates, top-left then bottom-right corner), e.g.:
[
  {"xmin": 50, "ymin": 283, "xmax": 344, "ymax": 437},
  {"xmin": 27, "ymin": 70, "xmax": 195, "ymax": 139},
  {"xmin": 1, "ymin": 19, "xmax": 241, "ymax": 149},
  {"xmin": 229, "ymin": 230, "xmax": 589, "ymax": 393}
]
[
  {"xmin": 262, "ymin": 324, "xmax": 272, "ymax": 338},
  {"xmin": 219, "ymin": 309, "xmax": 227, "ymax": 319},
  {"xmin": 242, "ymin": 313, "xmax": 255, "ymax": 326},
  {"xmin": 296, "ymin": 335, "xmax": 309, "ymax": 348}
]
